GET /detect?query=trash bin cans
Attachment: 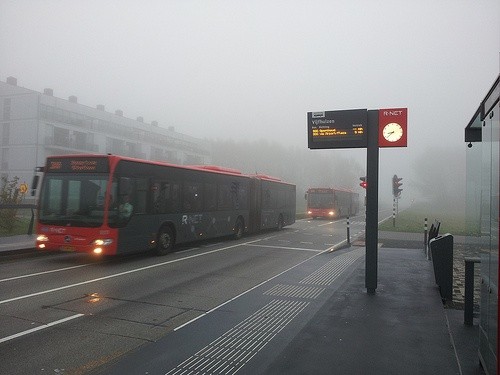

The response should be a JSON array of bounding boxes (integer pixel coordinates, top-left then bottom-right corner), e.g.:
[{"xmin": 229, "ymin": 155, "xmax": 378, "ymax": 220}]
[{"xmin": 429, "ymin": 233, "xmax": 453, "ymax": 301}]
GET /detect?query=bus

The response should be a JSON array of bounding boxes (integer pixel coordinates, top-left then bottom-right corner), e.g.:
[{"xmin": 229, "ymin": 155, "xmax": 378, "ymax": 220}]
[
  {"xmin": 304, "ymin": 187, "xmax": 360, "ymax": 219},
  {"xmin": 28, "ymin": 153, "xmax": 297, "ymax": 257}
]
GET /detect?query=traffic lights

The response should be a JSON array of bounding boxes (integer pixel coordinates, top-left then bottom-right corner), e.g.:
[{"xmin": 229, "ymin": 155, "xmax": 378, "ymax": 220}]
[
  {"xmin": 360, "ymin": 177, "xmax": 367, "ymax": 187},
  {"xmin": 392, "ymin": 174, "xmax": 403, "ymax": 197}
]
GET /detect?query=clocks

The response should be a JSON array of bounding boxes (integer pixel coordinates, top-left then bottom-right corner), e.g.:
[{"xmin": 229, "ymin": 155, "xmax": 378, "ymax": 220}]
[{"xmin": 383, "ymin": 122, "xmax": 403, "ymax": 142}]
